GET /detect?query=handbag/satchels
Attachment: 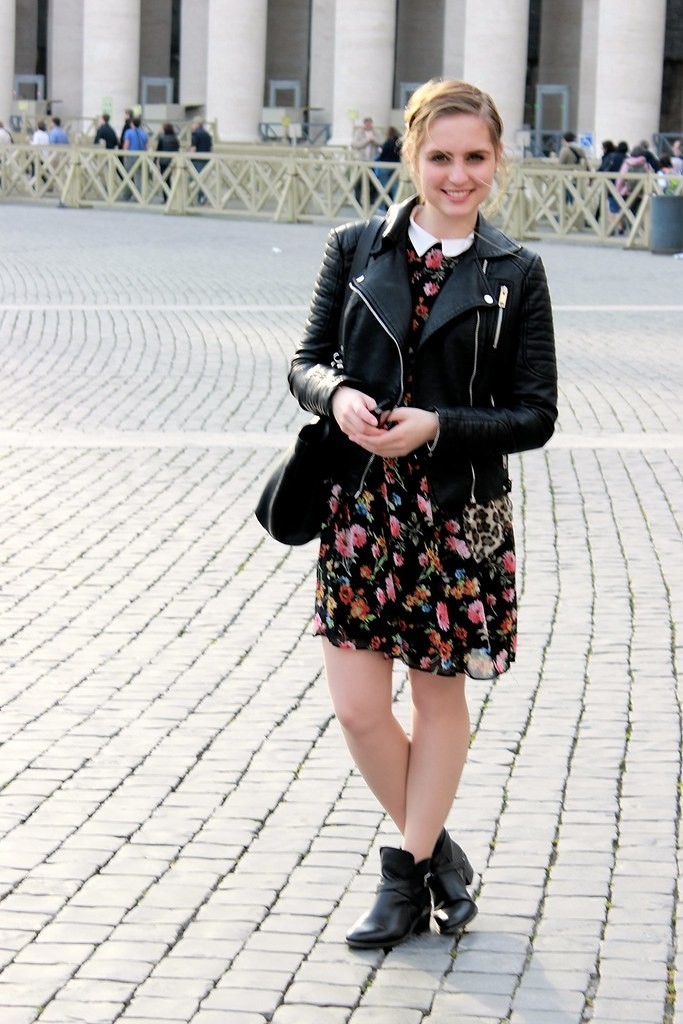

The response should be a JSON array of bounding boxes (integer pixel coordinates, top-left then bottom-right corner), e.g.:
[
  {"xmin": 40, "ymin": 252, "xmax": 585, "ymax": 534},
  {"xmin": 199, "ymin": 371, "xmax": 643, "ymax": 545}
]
[{"xmin": 256, "ymin": 418, "xmax": 342, "ymax": 548}]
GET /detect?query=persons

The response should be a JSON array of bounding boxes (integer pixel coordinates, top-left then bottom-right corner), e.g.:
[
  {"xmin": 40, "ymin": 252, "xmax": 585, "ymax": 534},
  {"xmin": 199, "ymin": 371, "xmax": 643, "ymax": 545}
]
[
  {"xmin": 639, "ymin": 140, "xmax": 659, "ymax": 172},
  {"xmin": 0, "ymin": 122, "xmax": 12, "ymax": 187},
  {"xmin": 152, "ymin": 123, "xmax": 180, "ymax": 203},
  {"xmin": 93, "ymin": 114, "xmax": 119, "ymax": 150},
  {"xmin": 115, "ymin": 107, "xmax": 134, "ymax": 182},
  {"xmin": 618, "ymin": 141, "xmax": 630, "ymax": 159},
  {"xmin": 25, "ymin": 120, "xmax": 49, "ymax": 190},
  {"xmin": 348, "ymin": 116, "xmax": 385, "ymax": 209},
  {"xmin": 121, "ymin": 118, "xmax": 149, "ymax": 202},
  {"xmin": 597, "ymin": 141, "xmax": 627, "ymax": 235},
  {"xmin": 373, "ymin": 126, "xmax": 403, "ymax": 215},
  {"xmin": 614, "ymin": 146, "xmax": 654, "ymax": 229},
  {"xmin": 46, "ymin": 118, "xmax": 68, "ymax": 192},
  {"xmin": 187, "ymin": 115, "xmax": 213, "ymax": 204},
  {"xmin": 285, "ymin": 78, "xmax": 559, "ymax": 950},
  {"xmin": 656, "ymin": 140, "xmax": 683, "ymax": 196},
  {"xmin": 557, "ymin": 132, "xmax": 594, "ymax": 203}
]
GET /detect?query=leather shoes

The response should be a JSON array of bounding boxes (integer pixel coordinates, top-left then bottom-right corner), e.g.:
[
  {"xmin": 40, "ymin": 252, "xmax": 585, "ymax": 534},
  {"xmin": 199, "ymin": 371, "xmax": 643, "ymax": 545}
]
[
  {"xmin": 424, "ymin": 826, "xmax": 479, "ymax": 935},
  {"xmin": 346, "ymin": 847, "xmax": 432, "ymax": 948}
]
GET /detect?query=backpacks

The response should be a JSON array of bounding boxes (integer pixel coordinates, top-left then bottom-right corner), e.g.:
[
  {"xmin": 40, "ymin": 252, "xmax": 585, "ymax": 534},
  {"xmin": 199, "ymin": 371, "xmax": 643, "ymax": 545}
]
[
  {"xmin": 568, "ymin": 146, "xmax": 587, "ymax": 180},
  {"xmin": 626, "ymin": 161, "xmax": 646, "ymax": 192}
]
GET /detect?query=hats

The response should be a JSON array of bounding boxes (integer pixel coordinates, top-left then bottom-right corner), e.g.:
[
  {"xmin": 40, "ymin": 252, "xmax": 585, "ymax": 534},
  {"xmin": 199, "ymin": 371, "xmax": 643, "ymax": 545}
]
[{"xmin": 562, "ymin": 132, "xmax": 576, "ymax": 142}]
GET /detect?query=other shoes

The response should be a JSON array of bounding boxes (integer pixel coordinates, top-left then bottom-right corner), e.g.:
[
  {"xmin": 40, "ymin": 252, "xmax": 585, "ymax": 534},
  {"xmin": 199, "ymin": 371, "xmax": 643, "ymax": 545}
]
[
  {"xmin": 618, "ymin": 228, "xmax": 623, "ymax": 234},
  {"xmin": 200, "ymin": 197, "xmax": 207, "ymax": 204},
  {"xmin": 611, "ymin": 230, "xmax": 615, "ymax": 236}
]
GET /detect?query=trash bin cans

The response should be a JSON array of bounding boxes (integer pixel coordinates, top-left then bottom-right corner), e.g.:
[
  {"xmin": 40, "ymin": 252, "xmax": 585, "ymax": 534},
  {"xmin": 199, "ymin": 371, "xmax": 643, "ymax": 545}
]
[{"xmin": 649, "ymin": 195, "xmax": 683, "ymax": 254}]
